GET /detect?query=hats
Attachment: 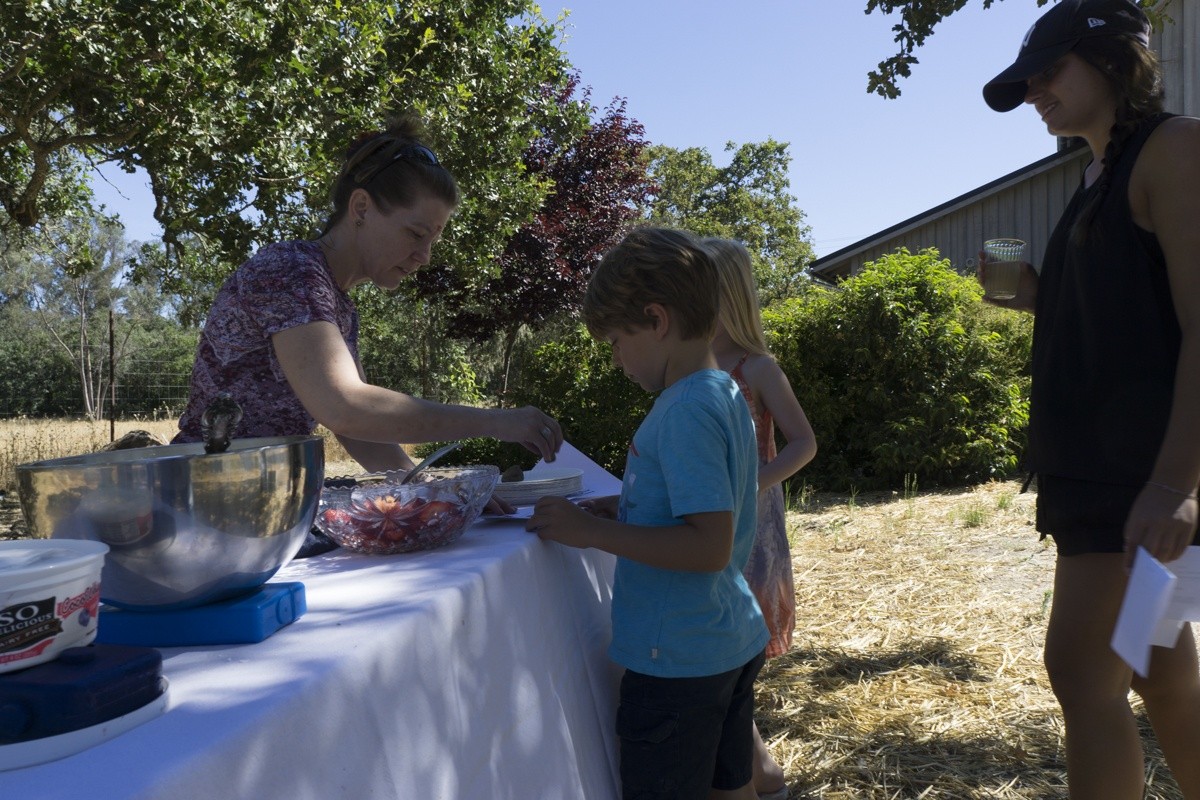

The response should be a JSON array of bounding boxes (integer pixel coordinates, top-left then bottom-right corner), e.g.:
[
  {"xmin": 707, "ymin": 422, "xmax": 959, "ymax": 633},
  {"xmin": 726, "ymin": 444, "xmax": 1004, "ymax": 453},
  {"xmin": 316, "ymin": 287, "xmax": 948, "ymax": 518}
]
[{"xmin": 983, "ymin": 0, "xmax": 1153, "ymax": 112}]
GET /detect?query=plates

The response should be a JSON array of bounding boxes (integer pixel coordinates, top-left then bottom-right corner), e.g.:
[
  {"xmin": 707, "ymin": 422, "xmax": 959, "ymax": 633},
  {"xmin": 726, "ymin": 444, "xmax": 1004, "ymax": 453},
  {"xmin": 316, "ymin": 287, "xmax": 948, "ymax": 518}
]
[{"xmin": 493, "ymin": 468, "xmax": 585, "ymax": 505}]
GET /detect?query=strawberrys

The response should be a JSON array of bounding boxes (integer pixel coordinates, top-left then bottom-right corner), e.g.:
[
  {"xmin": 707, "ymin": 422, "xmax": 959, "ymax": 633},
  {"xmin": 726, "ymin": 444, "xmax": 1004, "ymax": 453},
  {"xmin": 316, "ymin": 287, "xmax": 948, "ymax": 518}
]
[{"xmin": 319, "ymin": 496, "xmax": 465, "ymax": 551}]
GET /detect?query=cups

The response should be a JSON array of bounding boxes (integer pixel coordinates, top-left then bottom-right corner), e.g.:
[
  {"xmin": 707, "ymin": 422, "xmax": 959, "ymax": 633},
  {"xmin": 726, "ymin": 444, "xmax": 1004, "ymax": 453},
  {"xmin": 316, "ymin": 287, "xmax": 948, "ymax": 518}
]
[{"xmin": 982, "ymin": 238, "xmax": 1027, "ymax": 300}]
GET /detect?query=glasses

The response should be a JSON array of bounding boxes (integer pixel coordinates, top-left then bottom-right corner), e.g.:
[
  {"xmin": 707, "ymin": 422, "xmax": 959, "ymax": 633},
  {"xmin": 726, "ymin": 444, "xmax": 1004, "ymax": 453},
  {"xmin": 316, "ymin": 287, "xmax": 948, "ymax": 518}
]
[{"xmin": 361, "ymin": 144, "xmax": 445, "ymax": 189}]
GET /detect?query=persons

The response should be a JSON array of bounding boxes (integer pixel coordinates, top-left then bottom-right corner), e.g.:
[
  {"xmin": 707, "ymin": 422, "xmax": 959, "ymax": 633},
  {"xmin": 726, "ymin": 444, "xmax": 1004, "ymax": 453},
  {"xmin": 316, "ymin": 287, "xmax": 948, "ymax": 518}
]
[
  {"xmin": 525, "ymin": 227, "xmax": 773, "ymax": 799},
  {"xmin": 690, "ymin": 238, "xmax": 818, "ymax": 799},
  {"xmin": 979, "ymin": 1, "xmax": 1199, "ymax": 800},
  {"xmin": 168, "ymin": 118, "xmax": 563, "ymax": 517}
]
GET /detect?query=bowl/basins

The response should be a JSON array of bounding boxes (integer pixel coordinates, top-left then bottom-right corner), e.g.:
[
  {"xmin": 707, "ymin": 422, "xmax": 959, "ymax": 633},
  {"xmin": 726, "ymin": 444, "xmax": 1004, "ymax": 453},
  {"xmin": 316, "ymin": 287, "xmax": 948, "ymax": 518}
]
[
  {"xmin": 14, "ymin": 432, "xmax": 326, "ymax": 612},
  {"xmin": 314, "ymin": 465, "xmax": 500, "ymax": 555},
  {"xmin": 0, "ymin": 539, "xmax": 110, "ymax": 672}
]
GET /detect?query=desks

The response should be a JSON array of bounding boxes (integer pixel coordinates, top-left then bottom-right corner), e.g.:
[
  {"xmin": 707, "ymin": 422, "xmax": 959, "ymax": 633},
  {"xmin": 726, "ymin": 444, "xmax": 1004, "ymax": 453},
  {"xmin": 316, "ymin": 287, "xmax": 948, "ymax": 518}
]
[{"xmin": 0, "ymin": 485, "xmax": 624, "ymax": 800}]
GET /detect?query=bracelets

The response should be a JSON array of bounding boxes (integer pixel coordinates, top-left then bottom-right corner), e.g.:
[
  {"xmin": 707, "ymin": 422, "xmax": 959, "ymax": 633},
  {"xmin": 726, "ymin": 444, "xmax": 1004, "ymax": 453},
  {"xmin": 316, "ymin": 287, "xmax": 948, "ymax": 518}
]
[{"xmin": 1145, "ymin": 481, "xmax": 1198, "ymax": 502}]
